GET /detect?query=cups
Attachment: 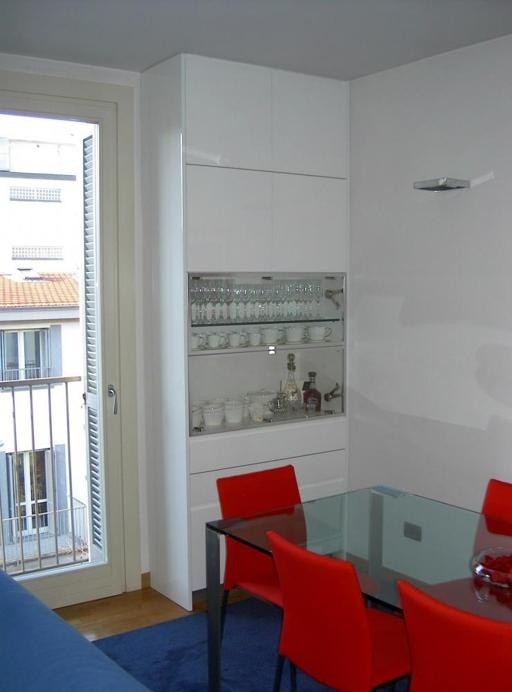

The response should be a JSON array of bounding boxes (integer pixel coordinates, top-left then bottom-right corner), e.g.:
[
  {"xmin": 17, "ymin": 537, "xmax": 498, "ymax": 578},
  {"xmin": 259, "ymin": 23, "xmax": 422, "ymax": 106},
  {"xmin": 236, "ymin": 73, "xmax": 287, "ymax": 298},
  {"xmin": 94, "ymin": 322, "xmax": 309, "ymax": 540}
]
[
  {"xmin": 190, "ymin": 393, "xmax": 276, "ymax": 428},
  {"xmin": 192, "ymin": 325, "xmax": 333, "ymax": 351}
]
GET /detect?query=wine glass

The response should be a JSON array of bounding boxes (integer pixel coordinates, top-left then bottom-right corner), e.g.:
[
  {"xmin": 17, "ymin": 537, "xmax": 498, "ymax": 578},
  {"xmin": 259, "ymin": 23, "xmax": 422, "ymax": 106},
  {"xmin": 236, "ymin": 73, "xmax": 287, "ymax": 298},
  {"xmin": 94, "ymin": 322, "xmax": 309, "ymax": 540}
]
[{"xmin": 193, "ymin": 279, "xmax": 325, "ymax": 325}]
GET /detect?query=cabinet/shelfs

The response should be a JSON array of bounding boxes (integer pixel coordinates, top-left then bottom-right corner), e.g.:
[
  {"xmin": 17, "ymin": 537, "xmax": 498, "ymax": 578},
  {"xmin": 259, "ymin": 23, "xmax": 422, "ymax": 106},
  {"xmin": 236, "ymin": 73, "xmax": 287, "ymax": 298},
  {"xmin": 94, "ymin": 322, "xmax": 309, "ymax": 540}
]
[
  {"xmin": 188, "ymin": 448, "xmax": 350, "ymax": 593},
  {"xmin": 186, "ymin": 275, "xmax": 348, "ymax": 435}
]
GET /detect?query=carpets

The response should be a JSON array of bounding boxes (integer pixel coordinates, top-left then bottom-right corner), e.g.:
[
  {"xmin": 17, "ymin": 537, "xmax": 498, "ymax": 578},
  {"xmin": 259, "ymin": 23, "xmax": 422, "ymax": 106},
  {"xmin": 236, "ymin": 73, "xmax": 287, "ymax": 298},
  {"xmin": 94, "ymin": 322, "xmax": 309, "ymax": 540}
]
[{"xmin": 86, "ymin": 595, "xmax": 413, "ymax": 692}]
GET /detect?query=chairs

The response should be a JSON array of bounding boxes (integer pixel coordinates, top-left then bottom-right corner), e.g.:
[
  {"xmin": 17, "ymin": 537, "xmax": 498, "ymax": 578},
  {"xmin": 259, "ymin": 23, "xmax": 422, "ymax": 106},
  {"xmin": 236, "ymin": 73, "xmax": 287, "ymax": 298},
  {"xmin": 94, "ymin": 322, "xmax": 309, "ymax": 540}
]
[
  {"xmin": 396, "ymin": 577, "xmax": 512, "ymax": 692},
  {"xmin": 216, "ymin": 463, "xmax": 299, "ymax": 692},
  {"xmin": 416, "ymin": 478, "xmax": 511, "ymax": 621},
  {"xmin": 266, "ymin": 531, "xmax": 413, "ymax": 690}
]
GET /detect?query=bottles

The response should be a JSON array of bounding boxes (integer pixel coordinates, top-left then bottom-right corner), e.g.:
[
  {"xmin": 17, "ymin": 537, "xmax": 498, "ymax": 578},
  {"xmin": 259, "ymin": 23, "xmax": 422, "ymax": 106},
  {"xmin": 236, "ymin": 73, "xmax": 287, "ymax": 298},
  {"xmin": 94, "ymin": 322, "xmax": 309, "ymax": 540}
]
[
  {"xmin": 303, "ymin": 372, "xmax": 322, "ymax": 412},
  {"xmin": 282, "ymin": 352, "xmax": 302, "ymax": 411}
]
